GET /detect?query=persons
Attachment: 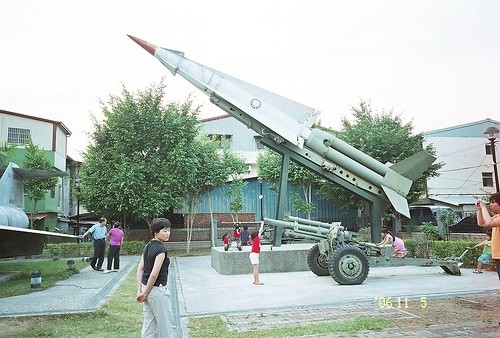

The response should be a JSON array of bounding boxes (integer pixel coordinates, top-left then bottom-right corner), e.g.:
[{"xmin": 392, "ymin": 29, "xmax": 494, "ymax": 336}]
[
  {"xmin": 475, "ymin": 192, "xmax": 500, "ymax": 280},
  {"xmin": 222, "ymin": 223, "xmax": 251, "ymax": 248},
  {"xmin": 377, "ymin": 228, "xmax": 406, "ymax": 259},
  {"xmin": 79, "ymin": 218, "xmax": 107, "ymax": 271},
  {"xmin": 249, "ymin": 220, "xmax": 265, "ymax": 285},
  {"xmin": 136, "ymin": 218, "xmax": 175, "ymax": 338},
  {"xmin": 473, "ymin": 232, "xmax": 492, "ymax": 274},
  {"xmin": 102, "ymin": 222, "xmax": 124, "ymax": 274}
]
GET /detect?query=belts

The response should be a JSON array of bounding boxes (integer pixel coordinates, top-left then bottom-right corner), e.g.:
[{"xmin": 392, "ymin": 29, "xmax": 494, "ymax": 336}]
[{"xmin": 98, "ymin": 238, "xmax": 105, "ymax": 240}]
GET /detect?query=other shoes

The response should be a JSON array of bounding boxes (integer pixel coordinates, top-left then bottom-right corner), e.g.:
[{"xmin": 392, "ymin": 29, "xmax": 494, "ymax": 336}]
[
  {"xmin": 97, "ymin": 268, "xmax": 104, "ymax": 271},
  {"xmin": 90, "ymin": 263, "xmax": 97, "ymax": 270},
  {"xmin": 111, "ymin": 269, "xmax": 119, "ymax": 272},
  {"xmin": 253, "ymin": 282, "xmax": 255, "ymax": 284},
  {"xmin": 103, "ymin": 270, "xmax": 111, "ymax": 273},
  {"xmin": 473, "ymin": 269, "xmax": 483, "ymax": 274},
  {"xmin": 255, "ymin": 281, "xmax": 264, "ymax": 285}
]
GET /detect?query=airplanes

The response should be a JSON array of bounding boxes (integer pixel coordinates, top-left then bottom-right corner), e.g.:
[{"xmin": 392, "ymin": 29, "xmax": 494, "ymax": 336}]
[{"xmin": 0, "ymin": 161, "xmax": 87, "ymax": 259}]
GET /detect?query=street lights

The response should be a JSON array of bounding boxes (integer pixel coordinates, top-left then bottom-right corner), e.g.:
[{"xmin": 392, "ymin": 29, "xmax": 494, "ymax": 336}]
[
  {"xmin": 483, "ymin": 126, "xmax": 500, "ymax": 192},
  {"xmin": 256, "ymin": 176, "xmax": 265, "ymax": 221}
]
